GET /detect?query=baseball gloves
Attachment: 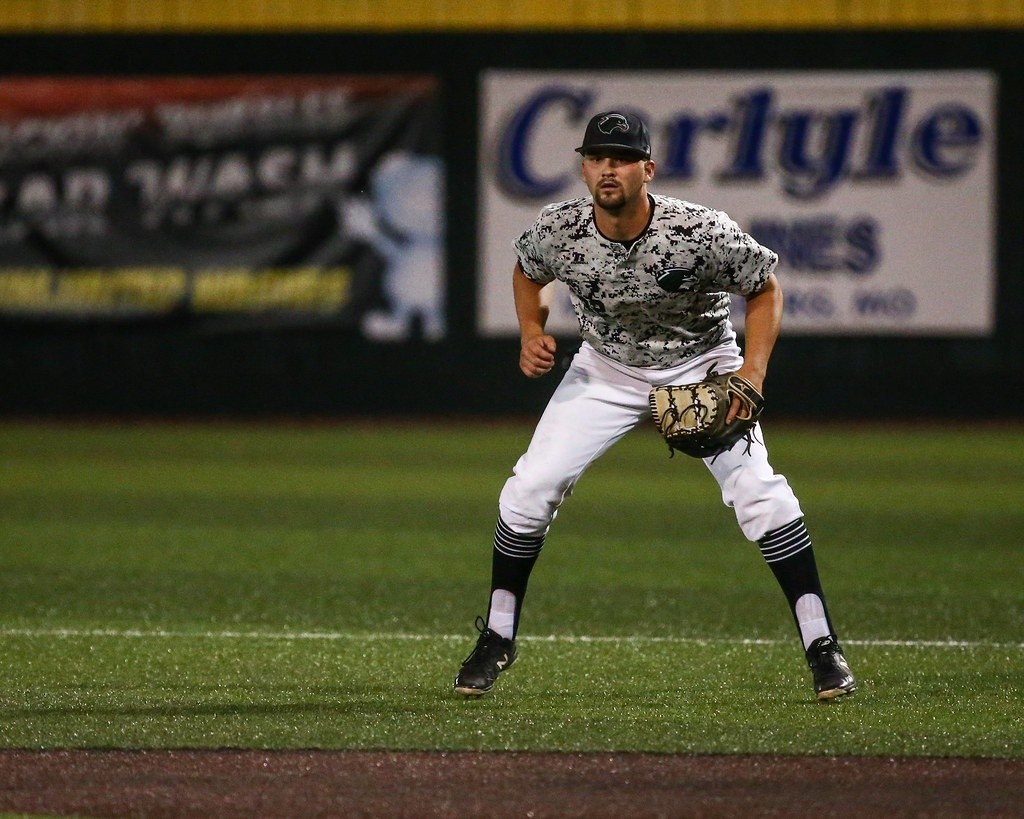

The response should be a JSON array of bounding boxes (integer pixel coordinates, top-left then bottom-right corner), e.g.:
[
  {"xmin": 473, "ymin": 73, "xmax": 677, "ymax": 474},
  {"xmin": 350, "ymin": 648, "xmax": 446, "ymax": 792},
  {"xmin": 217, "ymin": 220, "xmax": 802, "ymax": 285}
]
[{"xmin": 649, "ymin": 361, "xmax": 765, "ymax": 465}]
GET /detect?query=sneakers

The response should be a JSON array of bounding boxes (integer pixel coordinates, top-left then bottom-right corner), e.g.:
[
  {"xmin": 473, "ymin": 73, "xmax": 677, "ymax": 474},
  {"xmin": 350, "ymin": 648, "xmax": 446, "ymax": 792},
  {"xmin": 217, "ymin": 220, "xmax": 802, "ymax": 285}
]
[
  {"xmin": 806, "ymin": 634, "xmax": 856, "ymax": 700},
  {"xmin": 454, "ymin": 614, "xmax": 518, "ymax": 698}
]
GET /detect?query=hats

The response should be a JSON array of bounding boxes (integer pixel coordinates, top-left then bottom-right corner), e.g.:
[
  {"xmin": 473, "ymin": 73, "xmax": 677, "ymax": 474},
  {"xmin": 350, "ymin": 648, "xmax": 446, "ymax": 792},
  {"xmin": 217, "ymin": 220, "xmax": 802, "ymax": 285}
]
[{"xmin": 575, "ymin": 110, "xmax": 651, "ymax": 159}]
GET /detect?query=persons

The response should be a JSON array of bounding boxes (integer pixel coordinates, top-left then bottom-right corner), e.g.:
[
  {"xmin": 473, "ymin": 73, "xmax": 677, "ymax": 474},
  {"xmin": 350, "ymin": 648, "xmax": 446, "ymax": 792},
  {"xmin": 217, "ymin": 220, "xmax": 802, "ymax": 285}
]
[{"xmin": 454, "ymin": 110, "xmax": 858, "ymax": 699}]
